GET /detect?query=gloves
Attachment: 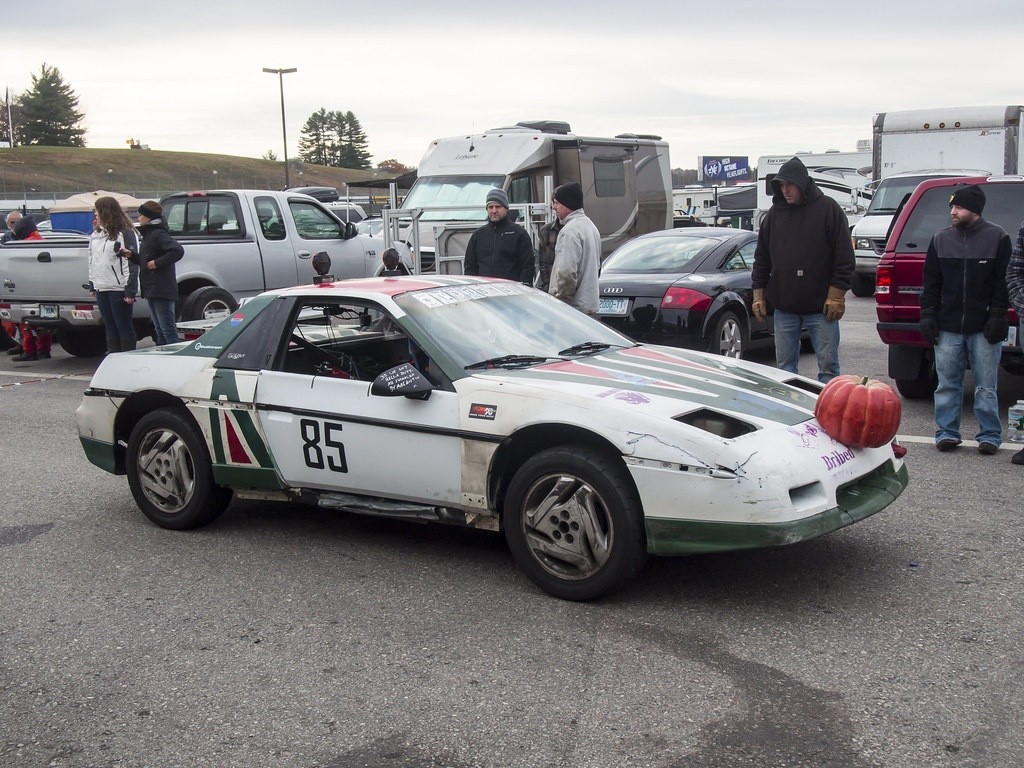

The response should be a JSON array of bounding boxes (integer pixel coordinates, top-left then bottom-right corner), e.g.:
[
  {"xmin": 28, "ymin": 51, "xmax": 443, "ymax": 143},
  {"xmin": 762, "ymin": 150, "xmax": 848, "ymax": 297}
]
[
  {"xmin": 752, "ymin": 288, "xmax": 768, "ymax": 320},
  {"xmin": 823, "ymin": 285, "xmax": 847, "ymax": 321},
  {"xmin": 919, "ymin": 309, "xmax": 939, "ymax": 345},
  {"xmin": 983, "ymin": 308, "xmax": 1008, "ymax": 344}
]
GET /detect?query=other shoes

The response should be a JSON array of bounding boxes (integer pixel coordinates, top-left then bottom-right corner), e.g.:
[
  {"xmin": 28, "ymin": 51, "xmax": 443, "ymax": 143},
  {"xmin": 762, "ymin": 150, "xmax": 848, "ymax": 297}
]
[
  {"xmin": 12, "ymin": 352, "xmax": 38, "ymax": 361},
  {"xmin": 937, "ymin": 439, "xmax": 956, "ymax": 451},
  {"xmin": 37, "ymin": 351, "xmax": 51, "ymax": 358},
  {"xmin": 1012, "ymin": 448, "xmax": 1024, "ymax": 464},
  {"xmin": 7, "ymin": 346, "xmax": 21, "ymax": 354},
  {"xmin": 977, "ymin": 442, "xmax": 996, "ymax": 454}
]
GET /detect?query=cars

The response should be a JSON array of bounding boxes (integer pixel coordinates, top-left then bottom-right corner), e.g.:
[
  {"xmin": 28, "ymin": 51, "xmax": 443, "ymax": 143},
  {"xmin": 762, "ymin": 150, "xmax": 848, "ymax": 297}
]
[
  {"xmin": 353, "ymin": 218, "xmax": 387, "ymax": 237},
  {"xmin": 598, "ymin": 226, "xmax": 815, "ymax": 358},
  {"xmin": 74, "ymin": 275, "xmax": 911, "ymax": 603}
]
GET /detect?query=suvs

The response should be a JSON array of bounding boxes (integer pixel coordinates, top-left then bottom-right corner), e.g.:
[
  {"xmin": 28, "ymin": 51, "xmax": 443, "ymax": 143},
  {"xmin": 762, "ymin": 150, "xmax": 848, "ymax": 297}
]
[
  {"xmin": 223, "ymin": 186, "xmax": 385, "ymax": 237},
  {"xmin": 873, "ymin": 174, "xmax": 1024, "ymax": 398}
]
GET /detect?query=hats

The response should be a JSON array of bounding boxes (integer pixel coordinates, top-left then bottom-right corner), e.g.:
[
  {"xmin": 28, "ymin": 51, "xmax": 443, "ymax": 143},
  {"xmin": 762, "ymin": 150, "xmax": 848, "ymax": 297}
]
[
  {"xmin": 13, "ymin": 214, "xmax": 37, "ymax": 239},
  {"xmin": 948, "ymin": 184, "xmax": 986, "ymax": 217},
  {"xmin": 137, "ymin": 201, "xmax": 163, "ymax": 220},
  {"xmin": 485, "ymin": 189, "xmax": 509, "ymax": 210},
  {"xmin": 553, "ymin": 182, "xmax": 583, "ymax": 211}
]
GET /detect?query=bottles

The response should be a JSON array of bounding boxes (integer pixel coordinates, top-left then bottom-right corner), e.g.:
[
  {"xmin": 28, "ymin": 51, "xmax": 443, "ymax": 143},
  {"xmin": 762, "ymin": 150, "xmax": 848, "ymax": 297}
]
[{"xmin": 1008, "ymin": 400, "xmax": 1024, "ymax": 444}]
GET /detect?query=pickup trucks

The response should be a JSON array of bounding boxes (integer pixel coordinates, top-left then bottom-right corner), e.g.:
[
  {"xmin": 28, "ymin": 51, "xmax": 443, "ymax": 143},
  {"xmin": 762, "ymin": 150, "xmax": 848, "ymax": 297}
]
[{"xmin": 0, "ymin": 187, "xmax": 415, "ymax": 357}]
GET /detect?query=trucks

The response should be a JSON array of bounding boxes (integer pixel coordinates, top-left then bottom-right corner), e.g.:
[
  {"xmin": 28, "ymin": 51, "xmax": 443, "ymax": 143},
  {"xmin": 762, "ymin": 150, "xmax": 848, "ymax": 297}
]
[
  {"xmin": 851, "ymin": 105, "xmax": 1023, "ymax": 297},
  {"xmin": 375, "ymin": 120, "xmax": 674, "ymax": 276},
  {"xmin": 673, "ymin": 145, "xmax": 874, "ymax": 233}
]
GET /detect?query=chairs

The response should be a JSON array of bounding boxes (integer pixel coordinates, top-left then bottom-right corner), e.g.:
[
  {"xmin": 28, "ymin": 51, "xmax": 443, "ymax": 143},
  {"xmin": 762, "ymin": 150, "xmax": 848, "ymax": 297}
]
[
  {"xmin": 210, "ymin": 215, "xmax": 228, "ymax": 229},
  {"xmin": 260, "ymin": 207, "xmax": 272, "ymax": 224}
]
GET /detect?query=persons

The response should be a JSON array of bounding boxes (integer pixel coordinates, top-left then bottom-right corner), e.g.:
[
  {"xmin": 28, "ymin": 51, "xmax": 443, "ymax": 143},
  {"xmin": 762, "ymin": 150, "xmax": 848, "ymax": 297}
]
[
  {"xmin": 751, "ymin": 157, "xmax": 857, "ymax": 384},
  {"xmin": 119, "ymin": 200, "xmax": 184, "ymax": 345},
  {"xmin": 89, "ymin": 197, "xmax": 140, "ymax": 352},
  {"xmin": 0, "ymin": 212, "xmax": 53, "ymax": 361},
  {"xmin": 919, "ymin": 184, "xmax": 1012, "ymax": 454},
  {"xmin": 538, "ymin": 182, "xmax": 602, "ymax": 318},
  {"xmin": 464, "ymin": 189, "xmax": 535, "ymax": 287},
  {"xmin": 1006, "ymin": 222, "xmax": 1024, "ymax": 464}
]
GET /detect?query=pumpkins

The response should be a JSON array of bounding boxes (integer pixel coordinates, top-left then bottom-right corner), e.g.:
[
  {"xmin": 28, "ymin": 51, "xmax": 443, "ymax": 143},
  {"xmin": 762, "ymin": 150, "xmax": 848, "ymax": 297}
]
[{"xmin": 814, "ymin": 375, "xmax": 901, "ymax": 448}]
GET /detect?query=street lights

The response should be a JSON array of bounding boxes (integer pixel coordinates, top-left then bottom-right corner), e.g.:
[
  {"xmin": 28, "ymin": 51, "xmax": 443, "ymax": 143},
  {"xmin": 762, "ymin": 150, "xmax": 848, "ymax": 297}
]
[{"xmin": 261, "ymin": 67, "xmax": 298, "ymax": 190}]
